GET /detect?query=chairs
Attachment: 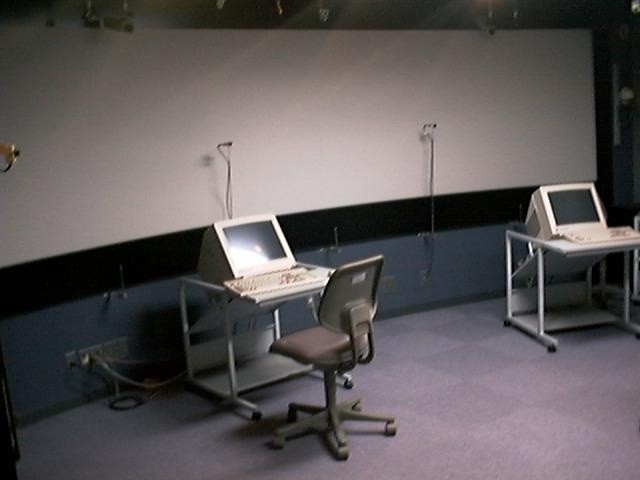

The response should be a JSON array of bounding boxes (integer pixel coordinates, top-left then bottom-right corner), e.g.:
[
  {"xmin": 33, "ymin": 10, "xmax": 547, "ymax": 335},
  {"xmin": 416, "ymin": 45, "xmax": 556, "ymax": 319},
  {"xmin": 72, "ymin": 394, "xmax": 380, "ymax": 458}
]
[{"xmin": 266, "ymin": 254, "xmax": 397, "ymax": 461}]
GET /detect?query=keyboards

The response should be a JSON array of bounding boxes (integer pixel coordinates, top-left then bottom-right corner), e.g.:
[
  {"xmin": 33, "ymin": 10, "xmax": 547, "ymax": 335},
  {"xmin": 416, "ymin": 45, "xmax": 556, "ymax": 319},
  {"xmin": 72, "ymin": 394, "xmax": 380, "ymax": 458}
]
[
  {"xmin": 224, "ymin": 267, "xmax": 323, "ymax": 294},
  {"xmin": 564, "ymin": 225, "xmax": 640, "ymax": 244}
]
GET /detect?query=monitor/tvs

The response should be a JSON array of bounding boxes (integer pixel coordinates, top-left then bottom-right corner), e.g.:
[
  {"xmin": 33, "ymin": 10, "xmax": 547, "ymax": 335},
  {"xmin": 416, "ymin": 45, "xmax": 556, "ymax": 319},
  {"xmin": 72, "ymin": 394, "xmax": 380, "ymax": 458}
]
[
  {"xmin": 198, "ymin": 214, "xmax": 297, "ymax": 284},
  {"xmin": 524, "ymin": 182, "xmax": 608, "ymax": 240}
]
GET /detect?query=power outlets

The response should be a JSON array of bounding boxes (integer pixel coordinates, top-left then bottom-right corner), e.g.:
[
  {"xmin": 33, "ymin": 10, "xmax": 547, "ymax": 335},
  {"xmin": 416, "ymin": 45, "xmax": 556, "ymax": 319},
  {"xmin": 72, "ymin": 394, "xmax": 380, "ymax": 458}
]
[{"xmin": 65, "ymin": 336, "xmax": 129, "ymax": 371}]
